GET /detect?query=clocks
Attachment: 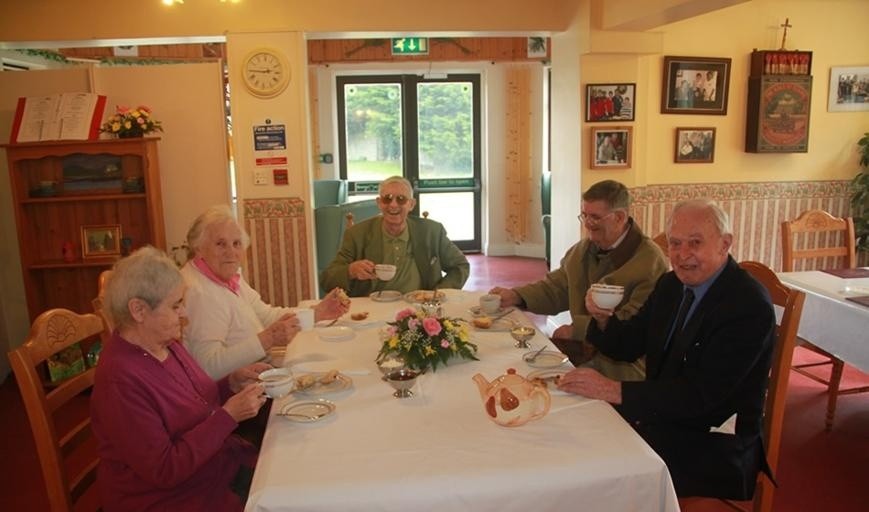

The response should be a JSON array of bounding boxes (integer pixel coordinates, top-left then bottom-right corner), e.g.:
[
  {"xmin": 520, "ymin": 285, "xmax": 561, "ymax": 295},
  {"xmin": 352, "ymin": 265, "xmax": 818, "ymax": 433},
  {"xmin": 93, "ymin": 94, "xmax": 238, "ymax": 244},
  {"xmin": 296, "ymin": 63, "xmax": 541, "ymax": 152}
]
[{"xmin": 242, "ymin": 47, "xmax": 292, "ymax": 100}]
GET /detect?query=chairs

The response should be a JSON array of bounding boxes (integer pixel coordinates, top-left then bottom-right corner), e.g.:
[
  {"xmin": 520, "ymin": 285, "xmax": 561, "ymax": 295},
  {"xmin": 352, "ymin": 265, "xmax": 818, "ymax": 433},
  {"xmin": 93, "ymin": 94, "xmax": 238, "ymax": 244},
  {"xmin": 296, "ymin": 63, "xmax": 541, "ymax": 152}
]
[
  {"xmin": 6, "ymin": 307, "xmax": 115, "ymax": 511},
  {"xmin": 651, "ymin": 232, "xmax": 670, "ymax": 259},
  {"xmin": 677, "ymin": 261, "xmax": 807, "ymax": 511},
  {"xmin": 781, "ymin": 207, "xmax": 869, "ymax": 432},
  {"xmin": 90, "ymin": 268, "xmax": 118, "ymax": 335}
]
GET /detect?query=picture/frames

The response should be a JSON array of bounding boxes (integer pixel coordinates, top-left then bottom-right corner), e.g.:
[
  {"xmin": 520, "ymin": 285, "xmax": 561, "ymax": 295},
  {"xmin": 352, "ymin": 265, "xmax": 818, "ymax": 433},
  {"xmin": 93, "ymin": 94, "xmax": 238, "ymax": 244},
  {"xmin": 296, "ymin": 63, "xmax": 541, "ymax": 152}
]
[
  {"xmin": 674, "ymin": 126, "xmax": 716, "ymax": 164},
  {"xmin": 660, "ymin": 54, "xmax": 732, "ymax": 116},
  {"xmin": 590, "ymin": 124, "xmax": 634, "ymax": 169},
  {"xmin": 827, "ymin": 65, "xmax": 869, "ymax": 113},
  {"xmin": 586, "ymin": 81, "xmax": 636, "ymax": 122},
  {"xmin": 80, "ymin": 224, "xmax": 123, "ymax": 261}
]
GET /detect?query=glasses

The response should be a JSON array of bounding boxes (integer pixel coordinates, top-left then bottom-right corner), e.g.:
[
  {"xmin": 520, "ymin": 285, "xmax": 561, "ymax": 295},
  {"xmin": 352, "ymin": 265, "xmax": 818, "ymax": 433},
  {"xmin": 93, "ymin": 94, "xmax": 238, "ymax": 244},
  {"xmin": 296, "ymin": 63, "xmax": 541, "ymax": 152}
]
[
  {"xmin": 577, "ymin": 211, "xmax": 614, "ymax": 224},
  {"xmin": 380, "ymin": 193, "xmax": 409, "ymax": 205}
]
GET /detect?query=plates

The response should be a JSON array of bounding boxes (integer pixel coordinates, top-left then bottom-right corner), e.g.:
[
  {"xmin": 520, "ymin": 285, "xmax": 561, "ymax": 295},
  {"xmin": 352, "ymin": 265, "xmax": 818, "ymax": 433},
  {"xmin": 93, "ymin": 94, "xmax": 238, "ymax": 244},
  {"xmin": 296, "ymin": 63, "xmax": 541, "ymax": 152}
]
[
  {"xmin": 521, "ymin": 351, "xmax": 570, "ymax": 367},
  {"xmin": 282, "ymin": 399, "xmax": 337, "ymax": 423},
  {"xmin": 403, "ymin": 289, "xmax": 446, "ymax": 305},
  {"xmin": 293, "ymin": 370, "xmax": 353, "ymax": 396},
  {"xmin": 527, "ymin": 370, "xmax": 582, "ymax": 396},
  {"xmin": 470, "ymin": 318, "xmax": 520, "ymax": 333},
  {"xmin": 468, "ymin": 305, "xmax": 510, "ymax": 318},
  {"xmin": 369, "ymin": 290, "xmax": 401, "ymax": 302},
  {"xmin": 317, "ymin": 325, "xmax": 353, "ymax": 342}
]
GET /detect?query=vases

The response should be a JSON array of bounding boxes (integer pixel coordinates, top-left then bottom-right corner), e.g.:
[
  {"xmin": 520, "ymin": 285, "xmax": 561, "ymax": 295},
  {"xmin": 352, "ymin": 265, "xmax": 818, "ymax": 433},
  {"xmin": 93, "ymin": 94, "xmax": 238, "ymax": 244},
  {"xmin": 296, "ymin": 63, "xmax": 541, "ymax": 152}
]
[{"xmin": 116, "ymin": 130, "xmax": 145, "ymax": 138}]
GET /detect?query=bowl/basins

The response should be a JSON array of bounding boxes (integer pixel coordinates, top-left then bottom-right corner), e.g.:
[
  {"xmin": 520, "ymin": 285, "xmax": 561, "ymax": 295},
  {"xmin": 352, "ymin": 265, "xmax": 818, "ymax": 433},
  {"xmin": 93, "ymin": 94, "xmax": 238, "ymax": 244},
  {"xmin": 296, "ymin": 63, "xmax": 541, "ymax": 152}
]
[
  {"xmin": 589, "ymin": 282, "xmax": 624, "ymax": 311},
  {"xmin": 480, "ymin": 294, "xmax": 501, "ymax": 315},
  {"xmin": 374, "ymin": 263, "xmax": 397, "ymax": 282}
]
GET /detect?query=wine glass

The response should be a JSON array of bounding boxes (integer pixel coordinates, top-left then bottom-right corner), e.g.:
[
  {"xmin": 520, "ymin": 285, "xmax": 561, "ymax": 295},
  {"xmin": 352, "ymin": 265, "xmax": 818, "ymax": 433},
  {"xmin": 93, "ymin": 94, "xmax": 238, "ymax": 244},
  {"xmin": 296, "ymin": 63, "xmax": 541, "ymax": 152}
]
[
  {"xmin": 509, "ymin": 326, "xmax": 536, "ymax": 349},
  {"xmin": 386, "ymin": 370, "xmax": 417, "ymax": 398}
]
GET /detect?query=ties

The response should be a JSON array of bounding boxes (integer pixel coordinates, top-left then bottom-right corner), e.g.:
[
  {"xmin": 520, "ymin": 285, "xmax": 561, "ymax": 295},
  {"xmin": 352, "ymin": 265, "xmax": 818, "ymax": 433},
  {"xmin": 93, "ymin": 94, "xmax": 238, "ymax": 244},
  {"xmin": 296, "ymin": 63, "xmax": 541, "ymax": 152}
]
[{"xmin": 668, "ymin": 287, "xmax": 695, "ymax": 346}]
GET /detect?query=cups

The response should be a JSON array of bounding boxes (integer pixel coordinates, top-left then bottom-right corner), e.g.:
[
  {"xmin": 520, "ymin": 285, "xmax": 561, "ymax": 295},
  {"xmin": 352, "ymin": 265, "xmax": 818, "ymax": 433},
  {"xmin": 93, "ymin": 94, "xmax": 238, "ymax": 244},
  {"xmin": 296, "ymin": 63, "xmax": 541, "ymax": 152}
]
[
  {"xmin": 258, "ymin": 367, "xmax": 294, "ymax": 400},
  {"xmin": 293, "ymin": 307, "xmax": 316, "ymax": 332}
]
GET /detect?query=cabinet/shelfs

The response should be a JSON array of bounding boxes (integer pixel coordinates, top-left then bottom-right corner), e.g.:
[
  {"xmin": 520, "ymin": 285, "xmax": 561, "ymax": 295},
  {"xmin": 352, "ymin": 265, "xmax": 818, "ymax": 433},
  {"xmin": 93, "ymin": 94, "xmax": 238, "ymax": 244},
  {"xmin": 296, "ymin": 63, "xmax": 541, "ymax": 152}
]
[{"xmin": 0, "ymin": 135, "xmax": 164, "ymax": 397}]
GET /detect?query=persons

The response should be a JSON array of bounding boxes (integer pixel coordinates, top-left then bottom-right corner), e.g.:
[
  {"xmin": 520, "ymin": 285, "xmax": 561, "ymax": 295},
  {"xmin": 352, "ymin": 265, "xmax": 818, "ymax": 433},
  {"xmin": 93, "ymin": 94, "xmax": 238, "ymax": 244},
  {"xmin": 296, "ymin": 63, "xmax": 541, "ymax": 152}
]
[
  {"xmin": 318, "ymin": 176, "xmax": 470, "ymax": 298},
  {"xmin": 598, "ymin": 135, "xmax": 617, "ymax": 160},
  {"xmin": 489, "ymin": 180, "xmax": 672, "ymax": 383},
  {"xmin": 89, "ymin": 246, "xmax": 273, "ymax": 512},
  {"xmin": 674, "ymin": 72, "xmax": 717, "ymax": 109},
  {"xmin": 556, "ymin": 199, "xmax": 780, "ymax": 501},
  {"xmin": 610, "ymin": 134, "xmax": 622, "ymax": 149},
  {"xmin": 176, "ymin": 204, "xmax": 351, "ymax": 428},
  {"xmin": 590, "ymin": 89, "xmax": 629, "ymax": 121},
  {"xmin": 681, "ymin": 131, "xmax": 713, "ymax": 159}
]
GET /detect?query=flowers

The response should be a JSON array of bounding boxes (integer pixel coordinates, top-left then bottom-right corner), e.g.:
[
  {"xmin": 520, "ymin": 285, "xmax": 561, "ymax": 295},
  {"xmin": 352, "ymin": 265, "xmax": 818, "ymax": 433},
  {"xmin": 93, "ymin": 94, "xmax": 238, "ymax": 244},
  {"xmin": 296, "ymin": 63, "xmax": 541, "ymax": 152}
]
[
  {"xmin": 372, "ymin": 301, "xmax": 480, "ymax": 374},
  {"xmin": 93, "ymin": 103, "xmax": 165, "ymax": 132}
]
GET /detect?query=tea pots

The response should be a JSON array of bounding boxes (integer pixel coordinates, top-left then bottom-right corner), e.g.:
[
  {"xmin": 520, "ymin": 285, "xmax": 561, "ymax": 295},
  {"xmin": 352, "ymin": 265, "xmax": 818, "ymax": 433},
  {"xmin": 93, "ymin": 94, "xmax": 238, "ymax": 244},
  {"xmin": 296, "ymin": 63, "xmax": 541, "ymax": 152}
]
[{"xmin": 470, "ymin": 367, "xmax": 553, "ymax": 429}]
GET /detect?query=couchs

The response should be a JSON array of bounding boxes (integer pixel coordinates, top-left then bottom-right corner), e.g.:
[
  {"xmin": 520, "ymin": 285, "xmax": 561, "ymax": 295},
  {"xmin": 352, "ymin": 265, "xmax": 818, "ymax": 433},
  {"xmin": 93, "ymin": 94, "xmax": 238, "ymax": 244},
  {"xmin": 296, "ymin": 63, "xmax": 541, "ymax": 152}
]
[
  {"xmin": 314, "ymin": 198, "xmax": 383, "ymax": 301},
  {"xmin": 314, "ymin": 179, "xmax": 348, "ymax": 207}
]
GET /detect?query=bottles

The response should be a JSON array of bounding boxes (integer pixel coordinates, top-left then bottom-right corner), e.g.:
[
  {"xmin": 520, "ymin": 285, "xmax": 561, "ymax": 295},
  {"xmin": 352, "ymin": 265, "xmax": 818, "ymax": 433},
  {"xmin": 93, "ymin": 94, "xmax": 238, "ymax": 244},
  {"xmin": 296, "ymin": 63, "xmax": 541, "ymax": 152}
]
[
  {"xmin": 123, "ymin": 238, "xmax": 138, "ymax": 256},
  {"xmin": 64, "ymin": 239, "xmax": 79, "ymax": 262}
]
